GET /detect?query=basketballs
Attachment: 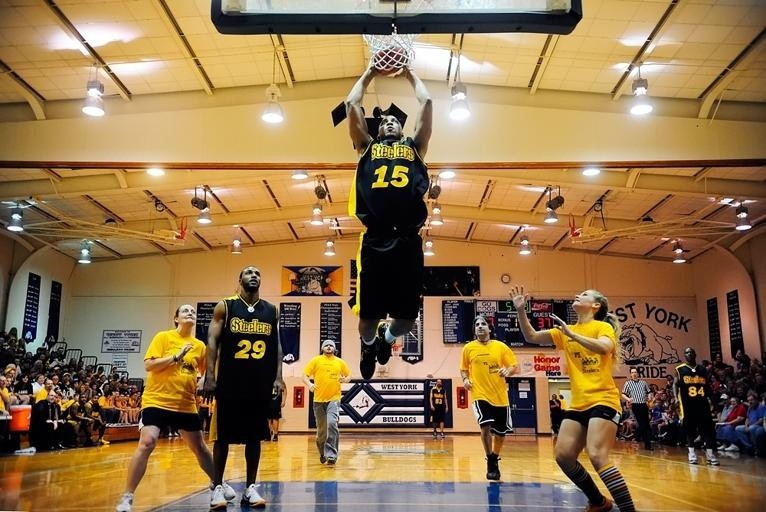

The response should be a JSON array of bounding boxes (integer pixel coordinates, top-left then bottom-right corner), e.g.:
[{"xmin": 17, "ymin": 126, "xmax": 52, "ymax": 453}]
[{"xmin": 374, "ymin": 45, "xmax": 409, "ymax": 74}]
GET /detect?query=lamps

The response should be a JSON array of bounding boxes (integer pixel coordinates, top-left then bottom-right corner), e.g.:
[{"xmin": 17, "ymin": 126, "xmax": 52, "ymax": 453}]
[
  {"xmin": 736, "ymin": 201, "xmax": 749, "ymax": 219},
  {"xmin": 7, "ymin": 201, "xmax": 26, "ymax": 232},
  {"xmin": 314, "ymin": 176, "xmax": 327, "ymax": 199},
  {"xmin": 231, "ymin": 224, "xmax": 243, "ymax": 257},
  {"xmin": 196, "ymin": 187, "xmax": 212, "ymax": 225},
  {"xmin": 673, "ymin": 240, "xmax": 684, "ymax": 255},
  {"xmin": 429, "ymin": 199, "xmax": 444, "ymax": 226},
  {"xmin": 78, "ymin": 61, "xmax": 106, "ymax": 119},
  {"xmin": 547, "ymin": 186, "xmax": 565, "ymax": 213},
  {"xmin": 594, "ymin": 198, "xmax": 603, "ymax": 211},
  {"xmin": 324, "ymin": 236, "xmax": 335, "ymax": 257},
  {"xmin": 260, "ymin": 46, "xmax": 285, "ymax": 124},
  {"xmin": 516, "ymin": 225, "xmax": 531, "ymax": 256},
  {"xmin": 445, "ymin": 43, "xmax": 471, "ymax": 125},
  {"xmin": 431, "ymin": 174, "xmax": 441, "ymax": 198},
  {"xmin": 669, "ymin": 237, "xmax": 688, "ymax": 264},
  {"xmin": 191, "ymin": 187, "xmax": 207, "ymax": 210},
  {"xmin": 155, "ymin": 199, "xmax": 165, "ymax": 212},
  {"xmin": 423, "ymin": 237, "xmax": 435, "ymax": 257},
  {"xmin": 734, "ymin": 197, "xmax": 754, "ymax": 232},
  {"xmin": 542, "ymin": 185, "xmax": 558, "ymax": 224},
  {"xmin": 76, "ymin": 240, "xmax": 94, "ymax": 264},
  {"xmin": 627, "ymin": 58, "xmax": 654, "ymax": 116},
  {"xmin": 311, "ymin": 198, "xmax": 323, "ymax": 228}
]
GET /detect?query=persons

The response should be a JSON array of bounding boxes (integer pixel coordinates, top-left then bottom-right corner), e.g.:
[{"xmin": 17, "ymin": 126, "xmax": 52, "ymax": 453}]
[
  {"xmin": 32, "ymin": 374, "xmax": 44, "ymax": 394},
  {"xmin": 559, "ymin": 395, "xmax": 567, "ymax": 411},
  {"xmin": 717, "ymin": 396, "xmax": 747, "ymax": 451},
  {"xmin": 714, "ymin": 394, "xmax": 733, "ymax": 428},
  {"xmin": 85, "ymin": 393, "xmax": 111, "ymax": 446},
  {"xmin": 69, "ymin": 394, "xmax": 100, "ymax": 447},
  {"xmin": 28, "ymin": 390, "xmax": 67, "ymax": 452},
  {"xmin": 98, "ymin": 375, "xmax": 142, "ymax": 424},
  {"xmin": 52, "ymin": 373, "xmax": 60, "ymax": 390},
  {"xmin": 549, "ymin": 393, "xmax": 563, "ymax": 434},
  {"xmin": 55, "ymin": 375, "xmax": 97, "ymax": 399},
  {"xmin": 673, "ymin": 348, "xmax": 719, "ymax": 464},
  {"xmin": 202, "ymin": 266, "xmax": 287, "ymax": 511},
  {"xmin": 264, "ymin": 375, "xmax": 287, "ymax": 441},
  {"xmin": 50, "ymin": 392, "xmax": 76, "ymax": 451},
  {"xmin": 507, "ymin": 283, "xmax": 637, "ymax": 512},
  {"xmin": 0, "ymin": 333, "xmax": 129, "ymax": 376},
  {"xmin": 702, "ymin": 350, "xmax": 766, "ymax": 405},
  {"xmin": 648, "ymin": 374, "xmax": 674, "ymax": 444},
  {"xmin": 735, "ymin": 391, "xmax": 766, "ymax": 457},
  {"xmin": 459, "ymin": 314, "xmax": 519, "ymax": 480},
  {"xmin": 615, "ymin": 400, "xmax": 635, "ymax": 440},
  {"xmin": 622, "ymin": 365, "xmax": 655, "ymax": 451},
  {"xmin": 197, "ymin": 392, "xmax": 216, "ymax": 433},
  {"xmin": 0, "ymin": 376, "xmax": 12, "ymax": 456},
  {"xmin": 35, "ymin": 378, "xmax": 55, "ymax": 404},
  {"xmin": 115, "ymin": 304, "xmax": 237, "ymax": 512},
  {"xmin": 344, "ymin": 55, "xmax": 433, "ymax": 379},
  {"xmin": 13, "ymin": 373, "xmax": 33, "ymax": 405},
  {"xmin": 303, "ymin": 341, "xmax": 352, "ymax": 465},
  {"xmin": 430, "ymin": 379, "xmax": 448, "ymax": 438}
]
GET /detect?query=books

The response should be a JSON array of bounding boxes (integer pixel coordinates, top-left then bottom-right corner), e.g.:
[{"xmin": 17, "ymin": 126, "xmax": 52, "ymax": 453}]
[{"xmin": 716, "ymin": 422, "xmax": 727, "ymax": 426}]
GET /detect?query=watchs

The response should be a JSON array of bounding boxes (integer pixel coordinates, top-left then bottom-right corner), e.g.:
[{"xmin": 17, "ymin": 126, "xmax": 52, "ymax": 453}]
[
  {"xmin": 463, "ymin": 377, "xmax": 468, "ymax": 381},
  {"xmin": 172, "ymin": 354, "xmax": 179, "ymax": 362}
]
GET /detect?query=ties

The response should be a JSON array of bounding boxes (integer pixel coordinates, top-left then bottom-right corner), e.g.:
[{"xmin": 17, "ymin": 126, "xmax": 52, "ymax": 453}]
[{"xmin": 51, "ymin": 405, "xmax": 55, "ymax": 430}]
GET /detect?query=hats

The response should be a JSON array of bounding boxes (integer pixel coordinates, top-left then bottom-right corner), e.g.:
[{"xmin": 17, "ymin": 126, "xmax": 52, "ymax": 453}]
[
  {"xmin": 322, "ymin": 340, "xmax": 336, "ymax": 350},
  {"xmin": 63, "ymin": 372, "xmax": 72, "ymax": 377},
  {"xmin": 5, "ymin": 367, "xmax": 16, "ymax": 374}
]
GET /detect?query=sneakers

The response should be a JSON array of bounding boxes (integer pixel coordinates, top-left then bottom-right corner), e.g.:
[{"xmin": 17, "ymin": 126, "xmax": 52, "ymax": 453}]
[
  {"xmin": 580, "ymin": 497, "xmax": 613, "ymax": 512},
  {"xmin": 717, "ymin": 444, "xmax": 728, "ymax": 450},
  {"xmin": 327, "ymin": 456, "xmax": 335, "ymax": 465},
  {"xmin": 84, "ymin": 437, "xmax": 93, "ymax": 446},
  {"xmin": 723, "ymin": 443, "xmax": 740, "ymax": 451},
  {"xmin": 377, "ymin": 321, "xmax": 393, "ymax": 365},
  {"xmin": 69, "ymin": 439, "xmax": 78, "ymax": 447},
  {"xmin": 211, "ymin": 480, "xmax": 236, "ymax": 499},
  {"xmin": 321, "ymin": 455, "xmax": 329, "ymax": 464},
  {"xmin": 362, "ymin": 341, "xmax": 377, "ymax": 378},
  {"xmin": 273, "ymin": 434, "xmax": 279, "ymax": 441},
  {"xmin": 266, "ymin": 435, "xmax": 272, "ymax": 441},
  {"xmin": 487, "ymin": 453, "xmax": 501, "ymax": 481},
  {"xmin": 91, "ymin": 437, "xmax": 100, "ymax": 445},
  {"xmin": 168, "ymin": 432, "xmax": 179, "ymax": 437},
  {"xmin": 241, "ymin": 485, "xmax": 267, "ymax": 508},
  {"xmin": 706, "ymin": 454, "xmax": 720, "ymax": 466},
  {"xmin": 209, "ymin": 486, "xmax": 228, "ymax": 510},
  {"xmin": 433, "ymin": 431, "xmax": 437, "ymax": 437},
  {"xmin": 97, "ymin": 437, "xmax": 110, "ymax": 444},
  {"xmin": 441, "ymin": 432, "xmax": 445, "ymax": 438},
  {"xmin": 689, "ymin": 452, "xmax": 698, "ymax": 464},
  {"xmin": 116, "ymin": 493, "xmax": 133, "ymax": 512}
]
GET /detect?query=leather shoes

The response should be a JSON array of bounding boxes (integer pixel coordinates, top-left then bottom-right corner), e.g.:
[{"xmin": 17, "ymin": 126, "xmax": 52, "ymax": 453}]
[
  {"xmin": 49, "ymin": 442, "xmax": 64, "ymax": 450},
  {"xmin": 645, "ymin": 444, "xmax": 653, "ymax": 450}
]
